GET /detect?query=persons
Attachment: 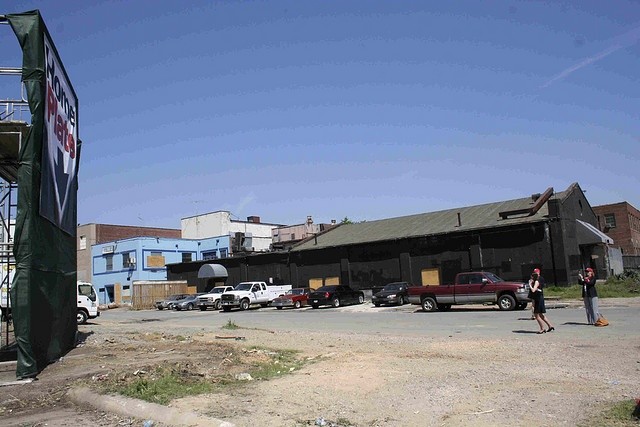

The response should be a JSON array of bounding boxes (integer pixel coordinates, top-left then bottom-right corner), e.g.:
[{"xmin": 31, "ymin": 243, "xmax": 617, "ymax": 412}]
[
  {"xmin": 527, "ymin": 268, "xmax": 545, "ymax": 321},
  {"xmin": 578, "ymin": 267, "xmax": 598, "ymax": 325},
  {"xmin": 528, "ymin": 273, "xmax": 554, "ymax": 334}
]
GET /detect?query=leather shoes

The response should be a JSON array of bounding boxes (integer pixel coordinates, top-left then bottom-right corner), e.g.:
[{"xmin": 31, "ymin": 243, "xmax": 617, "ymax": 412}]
[
  {"xmin": 536, "ymin": 329, "xmax": 546, "ymax": 334},
  {"xmin": 547, "ymin": 326, "xmax": 555, "ymax": 332}
]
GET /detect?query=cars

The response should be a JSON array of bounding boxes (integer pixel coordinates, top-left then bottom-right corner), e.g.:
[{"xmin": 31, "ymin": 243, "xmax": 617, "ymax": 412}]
[
  {"xmin": 271, "ymin": 287, "xmax": 316, "ymax": 309},
  {"xmin": 307, "ymin": 284, "xmax": 364, "ymax": 308},
  {"xmin": 371, "ymin": 281, "xmax": 414, "ymax": 306},
  {"xmin": 155, "ymin": 294, "xmax": 189, "ymax": 309},
  {"xmin": 172, "ymin": 293, "xmax": 204, "ymax": 310}
]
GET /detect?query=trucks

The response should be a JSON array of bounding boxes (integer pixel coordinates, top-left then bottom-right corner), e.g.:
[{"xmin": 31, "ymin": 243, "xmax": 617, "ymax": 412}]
[{"xmin": 0, "ymin": 257, "xmax": 99, "ymax": 324}]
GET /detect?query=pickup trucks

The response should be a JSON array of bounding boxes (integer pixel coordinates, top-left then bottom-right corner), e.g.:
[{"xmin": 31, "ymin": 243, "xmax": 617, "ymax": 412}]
[
  {"xmin": 196, "ymin": 285, "xmax": 234, "ymax": 310},
  {"xmin": 408, "ymin": 271, "xmax": 530, "ymax": 310},
  {"xmin": 220, "ymin": 281, "xmax": 293, "ymax": 311}
]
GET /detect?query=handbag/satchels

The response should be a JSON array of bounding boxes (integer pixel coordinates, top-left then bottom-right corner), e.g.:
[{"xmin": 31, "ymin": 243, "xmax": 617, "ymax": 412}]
[{"xmin": 595, "ymin": 312, "xmax": 609, "ymax": 326}]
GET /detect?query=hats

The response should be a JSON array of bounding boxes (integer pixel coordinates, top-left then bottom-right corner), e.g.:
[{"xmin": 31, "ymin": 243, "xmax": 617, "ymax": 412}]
[
  {"xmin": 584, "ymin": 267, "xmax": 593, "ymax": 272},
  {"xmin": 534, "ymin": 268, "xmax": 540, "ymax": 274}
]
